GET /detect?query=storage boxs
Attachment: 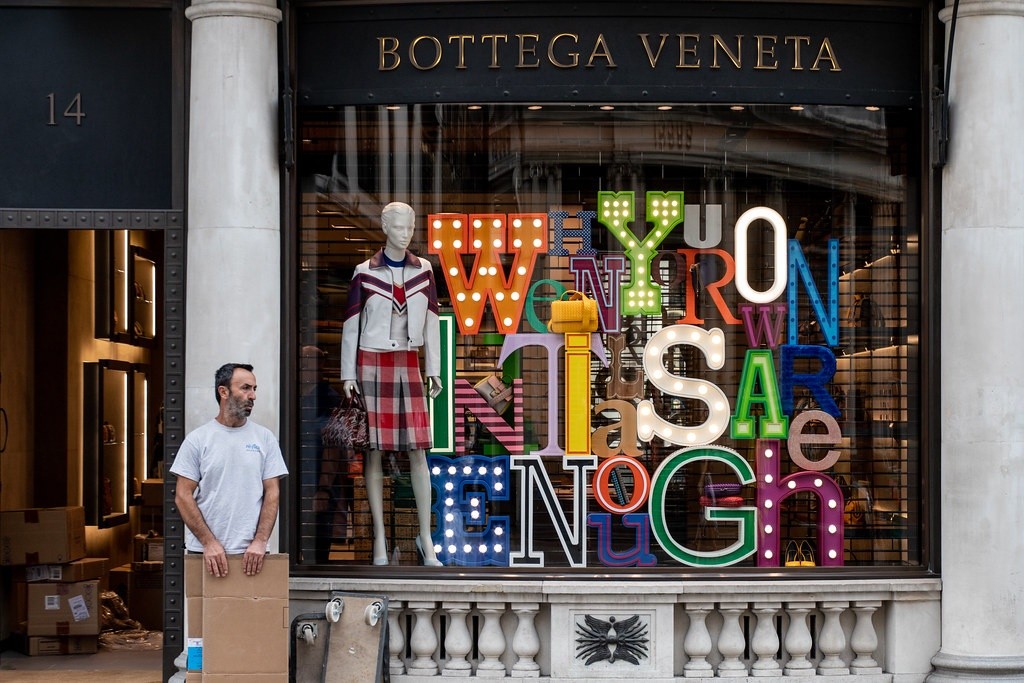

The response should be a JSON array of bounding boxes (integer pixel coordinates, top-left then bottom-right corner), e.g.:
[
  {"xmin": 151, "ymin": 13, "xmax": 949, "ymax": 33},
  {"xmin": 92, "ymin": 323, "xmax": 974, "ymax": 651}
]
[{"xmin": 0, "ymin": 504, "xmax": 111, "ymax": 657}]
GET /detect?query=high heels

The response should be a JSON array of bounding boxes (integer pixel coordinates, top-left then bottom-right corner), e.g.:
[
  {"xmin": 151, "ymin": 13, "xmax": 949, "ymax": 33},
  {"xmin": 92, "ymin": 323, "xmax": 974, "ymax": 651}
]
[
  {"xmin": 415, "ymin": 532, "xmax": 444, "ymax": 566},
  {"xmin": 373, "ymin": 538, "xmax": 389, "ymax": 566}
]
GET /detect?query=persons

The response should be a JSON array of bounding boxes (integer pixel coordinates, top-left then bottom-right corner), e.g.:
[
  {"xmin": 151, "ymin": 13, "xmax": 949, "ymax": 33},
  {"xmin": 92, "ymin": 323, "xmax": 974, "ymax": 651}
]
[
  {"xmin": 342, "ymin": 201, "xmax": 444, "ymax": 566},
  {"xmin": 170, "ymin": 363, "xmax": 289, "ymax": 578}
]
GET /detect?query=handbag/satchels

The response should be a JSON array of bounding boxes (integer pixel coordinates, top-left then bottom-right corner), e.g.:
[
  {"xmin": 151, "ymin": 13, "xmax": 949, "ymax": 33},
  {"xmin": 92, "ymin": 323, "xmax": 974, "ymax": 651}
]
[
  {"xmin": 320, "ymin": 388, "xmax": 369, "ymax": 450},
  {"xmin": 547, "ymin": 290, "xmax": 598, "ymax": 333},
  {"xmin": 690, "ymin": 263, "xmax": 712, "ymax": 286},
  {"xmin": 473, "ymin": 375, "xmax": 513, "ymax": 415}
]
